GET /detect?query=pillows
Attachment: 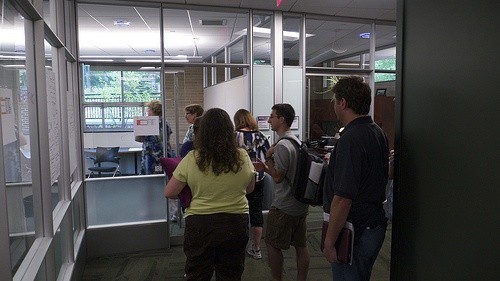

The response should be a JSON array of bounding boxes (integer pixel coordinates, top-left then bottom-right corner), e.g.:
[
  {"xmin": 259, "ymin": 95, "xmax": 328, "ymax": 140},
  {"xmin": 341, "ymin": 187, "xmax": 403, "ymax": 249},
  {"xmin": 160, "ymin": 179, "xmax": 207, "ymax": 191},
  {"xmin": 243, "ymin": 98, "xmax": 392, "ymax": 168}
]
[{"xmin": 160, "ymin": 157, "xmax": 192, "ymax": 211}]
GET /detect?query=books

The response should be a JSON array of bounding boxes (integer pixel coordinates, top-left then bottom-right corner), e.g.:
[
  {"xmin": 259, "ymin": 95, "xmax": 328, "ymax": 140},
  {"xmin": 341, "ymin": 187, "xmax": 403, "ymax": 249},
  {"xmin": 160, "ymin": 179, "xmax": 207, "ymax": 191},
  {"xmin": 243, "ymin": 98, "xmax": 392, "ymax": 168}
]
[{"xmin": 320, "ymin": 212, "xmax": 354, "ymax": 266}]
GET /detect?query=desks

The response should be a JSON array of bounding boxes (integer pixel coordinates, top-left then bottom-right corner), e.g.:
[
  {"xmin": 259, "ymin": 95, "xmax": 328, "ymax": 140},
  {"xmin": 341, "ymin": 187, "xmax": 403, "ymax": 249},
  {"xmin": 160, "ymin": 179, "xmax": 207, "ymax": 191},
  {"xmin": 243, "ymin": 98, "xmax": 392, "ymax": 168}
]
[{"xmin": 84, "ymin": 148, "xmax": 143, "ymax": 175}]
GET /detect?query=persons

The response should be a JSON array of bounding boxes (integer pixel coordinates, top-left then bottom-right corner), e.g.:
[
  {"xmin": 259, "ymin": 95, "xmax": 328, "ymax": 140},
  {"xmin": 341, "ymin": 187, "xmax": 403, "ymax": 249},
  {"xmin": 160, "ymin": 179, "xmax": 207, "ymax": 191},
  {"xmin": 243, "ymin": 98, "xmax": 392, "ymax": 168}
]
[
  {"xmin": 164, "ymin": 108, "xmax": 256, "ymax": 281},
  {"xmin": 233, "ymin": 108, "xmax": 274, "ymax": 259},
  {"xmin": 321, "ymin": 77, "xmax": 389, "ymax": 281},
  {"xmin": 252, "ymin": 103, "xmax": 310, "ymax": 281},
  {"xmin": 136, "ymin": 100, "xmax": 206, "ymax": 222}
]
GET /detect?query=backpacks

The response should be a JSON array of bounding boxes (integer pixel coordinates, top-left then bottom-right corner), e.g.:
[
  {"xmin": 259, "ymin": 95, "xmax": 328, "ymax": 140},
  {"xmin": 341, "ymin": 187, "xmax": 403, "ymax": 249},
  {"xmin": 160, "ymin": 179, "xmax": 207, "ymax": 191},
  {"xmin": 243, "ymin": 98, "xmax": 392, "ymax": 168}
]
[{"xmin": 273, "ymin": 136, "xmax": 326, "ymax": 206}]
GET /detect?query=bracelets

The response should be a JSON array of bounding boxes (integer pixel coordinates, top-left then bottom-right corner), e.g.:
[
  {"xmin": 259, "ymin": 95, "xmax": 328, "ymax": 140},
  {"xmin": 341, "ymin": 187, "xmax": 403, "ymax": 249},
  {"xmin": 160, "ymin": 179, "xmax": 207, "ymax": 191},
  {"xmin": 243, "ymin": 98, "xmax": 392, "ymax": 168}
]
[{"xmin": 266, "ymin": 156, "xmax": 272, "ymax": 160}]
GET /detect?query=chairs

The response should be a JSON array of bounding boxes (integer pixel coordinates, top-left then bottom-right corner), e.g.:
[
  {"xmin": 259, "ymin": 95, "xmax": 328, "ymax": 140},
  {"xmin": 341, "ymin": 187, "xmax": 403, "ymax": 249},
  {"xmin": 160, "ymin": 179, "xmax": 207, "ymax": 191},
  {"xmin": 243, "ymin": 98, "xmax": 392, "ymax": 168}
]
[{"xmin": 88, "ymin": 147, "xmax": 122, "ymax": 178}]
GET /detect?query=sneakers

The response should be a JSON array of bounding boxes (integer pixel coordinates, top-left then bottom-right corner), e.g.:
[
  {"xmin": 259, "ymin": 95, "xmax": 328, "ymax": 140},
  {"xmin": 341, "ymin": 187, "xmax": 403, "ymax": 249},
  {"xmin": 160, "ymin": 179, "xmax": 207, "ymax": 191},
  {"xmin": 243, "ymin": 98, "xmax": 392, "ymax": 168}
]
[{"xmin": 246, "ymin": 245, "xmax": 263, "ymax": 259}]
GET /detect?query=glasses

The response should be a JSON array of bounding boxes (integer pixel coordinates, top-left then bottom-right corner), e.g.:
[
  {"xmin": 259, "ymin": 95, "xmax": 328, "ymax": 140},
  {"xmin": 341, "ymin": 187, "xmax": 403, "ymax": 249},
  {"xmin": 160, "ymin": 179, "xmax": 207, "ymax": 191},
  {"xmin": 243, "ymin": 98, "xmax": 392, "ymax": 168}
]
[{"xmin": 268, "ymin": 113, "xmax": 284, "ymax": 119}]
